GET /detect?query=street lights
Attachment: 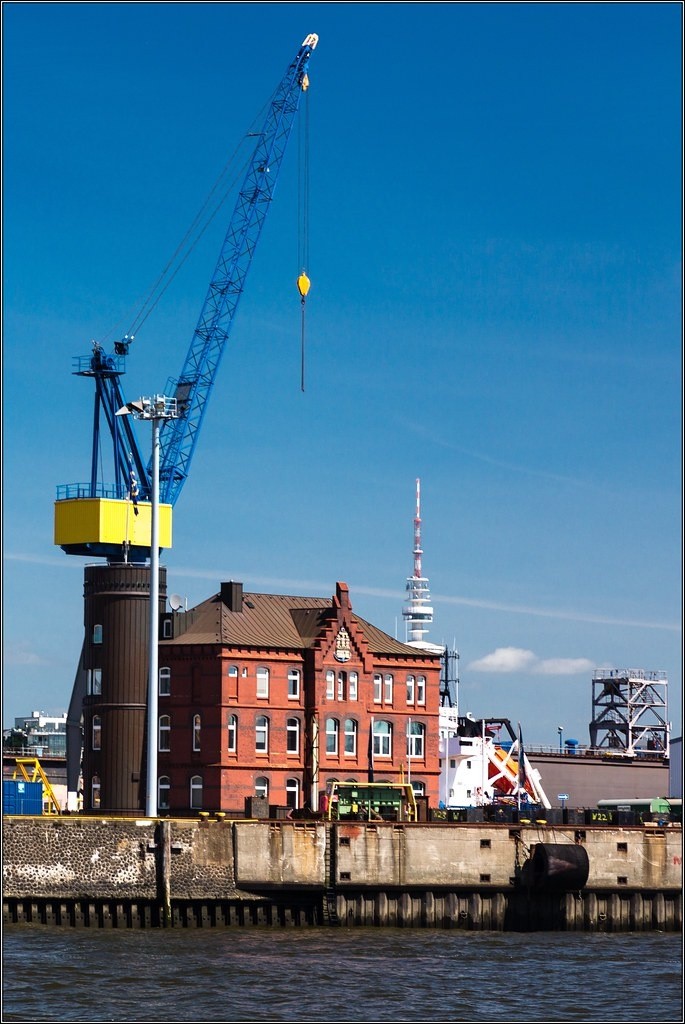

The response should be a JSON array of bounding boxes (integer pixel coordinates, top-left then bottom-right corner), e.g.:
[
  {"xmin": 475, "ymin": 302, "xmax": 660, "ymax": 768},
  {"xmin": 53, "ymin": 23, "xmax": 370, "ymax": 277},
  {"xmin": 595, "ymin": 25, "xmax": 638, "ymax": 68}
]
[{"xmin": 557, "ymin": 726, "xmax": 563, "ymax": 753}]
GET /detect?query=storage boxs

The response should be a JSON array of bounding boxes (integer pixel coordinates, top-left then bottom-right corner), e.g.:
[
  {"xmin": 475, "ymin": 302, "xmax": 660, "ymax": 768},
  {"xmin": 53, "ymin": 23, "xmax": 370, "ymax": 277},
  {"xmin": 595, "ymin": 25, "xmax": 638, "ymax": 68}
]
[{"xmin": 3, "ymin": 781, "xmax": 42, "ymax": 815}]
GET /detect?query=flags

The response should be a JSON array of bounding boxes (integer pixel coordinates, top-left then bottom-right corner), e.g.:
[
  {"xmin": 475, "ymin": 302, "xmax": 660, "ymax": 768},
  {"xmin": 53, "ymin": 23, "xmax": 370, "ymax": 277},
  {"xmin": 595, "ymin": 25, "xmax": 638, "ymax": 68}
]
[{"xmin": 127, "ymin": 461, "xmax": 140, "ymax": 515}]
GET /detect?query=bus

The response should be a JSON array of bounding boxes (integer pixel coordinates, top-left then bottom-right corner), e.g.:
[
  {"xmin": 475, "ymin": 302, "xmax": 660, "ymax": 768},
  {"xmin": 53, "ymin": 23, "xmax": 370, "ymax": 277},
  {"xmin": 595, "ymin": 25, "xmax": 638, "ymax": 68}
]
[{"xmin": 598, "ymin": 798, "xmax": 682, "ymax": 828}]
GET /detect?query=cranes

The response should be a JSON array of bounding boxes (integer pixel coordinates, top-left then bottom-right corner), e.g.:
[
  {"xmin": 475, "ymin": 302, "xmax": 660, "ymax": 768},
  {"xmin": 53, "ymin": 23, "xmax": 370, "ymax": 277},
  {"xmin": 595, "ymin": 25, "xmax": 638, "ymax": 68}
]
[{"xmin": 51, "ymin": 33, "xmax": 323, "ymax": 561}]
